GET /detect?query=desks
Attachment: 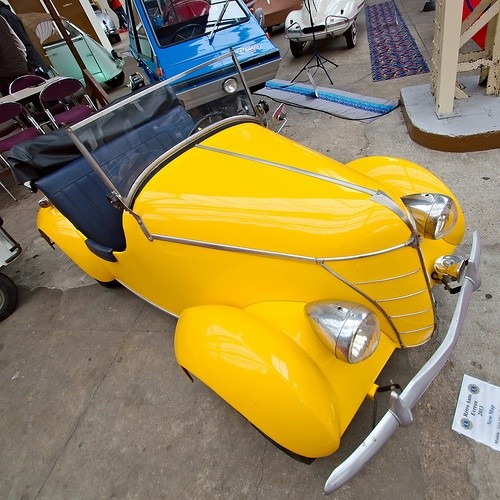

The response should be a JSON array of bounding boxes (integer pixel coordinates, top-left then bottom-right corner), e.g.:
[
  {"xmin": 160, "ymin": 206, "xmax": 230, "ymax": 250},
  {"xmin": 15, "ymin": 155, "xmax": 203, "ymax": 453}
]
[{"xmin": 0, "ymin": 76, "xmax": 74, "ymax": 103}]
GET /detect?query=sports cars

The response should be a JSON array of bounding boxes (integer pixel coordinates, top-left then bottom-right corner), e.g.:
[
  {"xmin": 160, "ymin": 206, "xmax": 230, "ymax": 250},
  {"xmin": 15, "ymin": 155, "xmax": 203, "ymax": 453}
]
[
  {"xmin": 7, "ymin": 46, "xmax": 482, "ymax": 496},
  {"xmin": 90, "ymin": 3, "xmax": 122, "ymax": 45},
  {"xmin": 285, "ymin": 0, "xmax": 367, "ymax": 59}
]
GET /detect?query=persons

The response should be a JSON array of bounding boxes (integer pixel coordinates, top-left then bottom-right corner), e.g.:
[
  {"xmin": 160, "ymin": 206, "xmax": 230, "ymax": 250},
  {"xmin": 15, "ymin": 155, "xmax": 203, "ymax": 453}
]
[
  {"xmin": 0, "ymin": 0, "xmax": 66, "ymax": 134},
  {"xmin": 107, "ymin": 0, "xmax": 128, "ymax": 30}
]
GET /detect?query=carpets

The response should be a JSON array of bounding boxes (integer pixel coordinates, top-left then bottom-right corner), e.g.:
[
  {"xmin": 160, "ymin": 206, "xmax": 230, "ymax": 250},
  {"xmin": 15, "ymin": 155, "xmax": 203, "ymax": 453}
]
[{"xmin": 364, "ymin": 1, "xmax": 430, "ymax": 83}]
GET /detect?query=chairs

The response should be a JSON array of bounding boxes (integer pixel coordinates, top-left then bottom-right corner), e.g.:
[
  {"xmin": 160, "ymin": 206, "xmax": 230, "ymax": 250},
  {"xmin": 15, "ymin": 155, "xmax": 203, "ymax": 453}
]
[
  {"xmin": 0, "ymin": 182, "xmax": 19, "ymax": 203},
  {"xmin": 39, "ymin": 77, "xmax": 98, "ymax": 131},
  {"xmin": 0, "ymin": 101, "xmax": 46, "ymax": 185},
  {"xmin": 9, "ymin": 74, "xmax": 69, "ymax": 126}
]
